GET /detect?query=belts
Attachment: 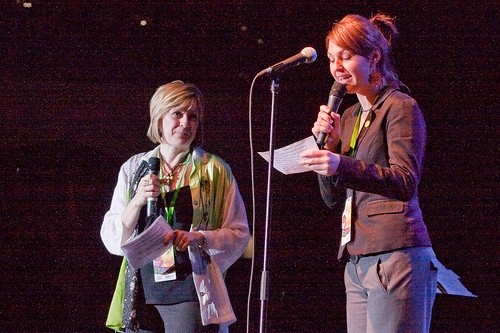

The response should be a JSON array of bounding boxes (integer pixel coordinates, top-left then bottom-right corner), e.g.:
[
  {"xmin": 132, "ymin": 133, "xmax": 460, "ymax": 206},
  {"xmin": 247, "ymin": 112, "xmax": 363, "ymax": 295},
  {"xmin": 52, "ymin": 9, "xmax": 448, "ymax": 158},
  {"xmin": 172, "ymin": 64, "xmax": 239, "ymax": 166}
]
[{"xmin": 347, "ymin": 254, "xmax": 368, "ymax": 264}]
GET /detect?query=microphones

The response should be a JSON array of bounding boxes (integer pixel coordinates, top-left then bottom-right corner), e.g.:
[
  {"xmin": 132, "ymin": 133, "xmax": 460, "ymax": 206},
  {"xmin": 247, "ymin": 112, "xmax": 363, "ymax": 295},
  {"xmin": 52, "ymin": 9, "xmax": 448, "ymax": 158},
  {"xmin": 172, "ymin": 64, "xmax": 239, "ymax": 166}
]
[
  {"xmin": 317, "ymin": 82, "xmax": 347, "ymax": 145},
  {"xmin": 146, "ymin": 156, "xmax": 161, "ymax": 218},
  {"xmin": 257, "ymin": 47, "xmax": 317, "ymax": 77}
]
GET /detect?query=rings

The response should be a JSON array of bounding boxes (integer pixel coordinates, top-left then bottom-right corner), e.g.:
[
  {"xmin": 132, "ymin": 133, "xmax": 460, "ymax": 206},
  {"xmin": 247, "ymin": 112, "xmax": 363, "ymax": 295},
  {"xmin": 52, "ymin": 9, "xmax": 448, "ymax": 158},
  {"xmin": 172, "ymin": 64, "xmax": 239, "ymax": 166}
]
[{"xmin": 144, "ymin": 180, "xmax": 149, "ymax": 186}]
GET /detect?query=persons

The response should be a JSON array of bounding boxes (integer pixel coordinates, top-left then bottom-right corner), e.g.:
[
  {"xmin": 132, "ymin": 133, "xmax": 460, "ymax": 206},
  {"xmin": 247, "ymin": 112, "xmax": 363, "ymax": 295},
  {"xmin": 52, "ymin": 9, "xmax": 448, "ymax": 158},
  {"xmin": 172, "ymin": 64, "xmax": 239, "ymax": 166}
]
[
  {"xmin": 298, "ymin": 13, "xmax": 440, "ymax": 333},
  {"xmin": 99, "ymin": 78, "xmax": 250, "ymax": 333}
]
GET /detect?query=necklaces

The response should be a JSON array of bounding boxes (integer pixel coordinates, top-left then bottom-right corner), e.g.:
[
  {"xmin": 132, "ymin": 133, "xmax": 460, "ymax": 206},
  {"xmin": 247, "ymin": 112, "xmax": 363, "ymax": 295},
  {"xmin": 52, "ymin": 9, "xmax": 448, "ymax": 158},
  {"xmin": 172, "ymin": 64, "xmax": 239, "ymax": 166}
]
[
  {"xmin": 160, "ymin": 151, "xmax": 192, "ymax": 192},
  {"xmin": 360, "ymin": 85, "xmax": 388, "ymax": 112}
]
